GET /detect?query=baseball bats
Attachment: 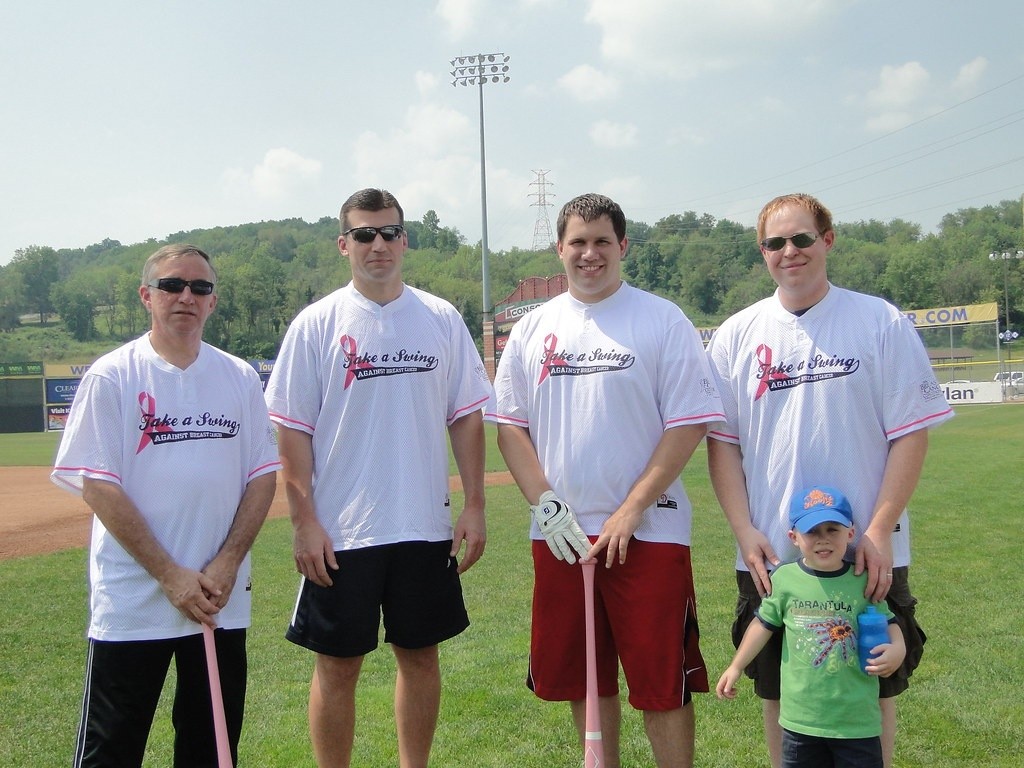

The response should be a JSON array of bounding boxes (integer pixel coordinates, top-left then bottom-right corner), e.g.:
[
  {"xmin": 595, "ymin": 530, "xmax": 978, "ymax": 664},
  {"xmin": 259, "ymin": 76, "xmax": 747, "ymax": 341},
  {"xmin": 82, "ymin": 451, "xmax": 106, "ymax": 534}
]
[
  {"xmin": 202, "ymin": 623, "xmax": 235, "ymax": 768},
  {"xmin": 579, "ymin": 557, "xmax": 605, "ymax": 768}
]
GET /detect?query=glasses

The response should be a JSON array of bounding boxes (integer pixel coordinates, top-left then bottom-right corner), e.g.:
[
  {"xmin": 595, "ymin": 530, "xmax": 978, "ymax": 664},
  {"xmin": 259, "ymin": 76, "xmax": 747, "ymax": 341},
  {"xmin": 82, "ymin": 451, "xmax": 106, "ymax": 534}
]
[
  {"xmin": 148, "ymin": 277, "xmax": 214, "ymax": 295},
  {"xmin": 760, "ymin": 231, "xmax": 820, "ymax": 252},
  {"xmin": 343, "ymin": 225, "xmax": 403, "ymax": 243}
]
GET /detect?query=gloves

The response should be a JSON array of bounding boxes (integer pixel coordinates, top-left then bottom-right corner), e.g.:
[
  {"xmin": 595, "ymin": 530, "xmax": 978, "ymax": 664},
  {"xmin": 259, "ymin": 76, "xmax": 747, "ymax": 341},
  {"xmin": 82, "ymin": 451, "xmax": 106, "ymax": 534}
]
[{"xmin": 530, "ymin": 489, "xmax": 593, "ymax": 565}]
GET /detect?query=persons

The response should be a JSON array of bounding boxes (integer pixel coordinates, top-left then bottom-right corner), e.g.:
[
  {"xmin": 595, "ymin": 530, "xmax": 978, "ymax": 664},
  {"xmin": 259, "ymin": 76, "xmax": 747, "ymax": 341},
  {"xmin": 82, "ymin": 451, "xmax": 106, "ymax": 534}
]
[
  {"xmin": 706, "ymin": 194, "xmax": 955, "ymax": 768},
  {"xmin": 481, "ymin": 192, "xmax": 727, "ymax": 768},
  {"xmin": 263, "ymin": 188, "xmax": 494, "ymax": 768},
  {"xmin": 50, "ymin": 246, "xmax": 283, "ymax": 768}
]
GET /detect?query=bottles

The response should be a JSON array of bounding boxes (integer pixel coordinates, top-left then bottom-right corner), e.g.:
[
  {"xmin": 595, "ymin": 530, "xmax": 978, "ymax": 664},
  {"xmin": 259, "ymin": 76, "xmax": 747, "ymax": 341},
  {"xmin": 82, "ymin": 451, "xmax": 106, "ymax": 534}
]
[{"xmin": 858, "ymin": 605, "xmax": 891, "ymax": 673}]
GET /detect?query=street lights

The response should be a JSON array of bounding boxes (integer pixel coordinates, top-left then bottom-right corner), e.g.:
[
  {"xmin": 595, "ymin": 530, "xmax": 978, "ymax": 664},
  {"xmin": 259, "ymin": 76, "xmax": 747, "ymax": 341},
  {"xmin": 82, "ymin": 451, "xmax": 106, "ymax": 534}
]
[
  {"xmin": 987, "ymin": 244, "xmax": 1022, "ymax": 395},
  {"xmin": 448, "ymin": 52, "xmax": 511, "ymax": 384}
]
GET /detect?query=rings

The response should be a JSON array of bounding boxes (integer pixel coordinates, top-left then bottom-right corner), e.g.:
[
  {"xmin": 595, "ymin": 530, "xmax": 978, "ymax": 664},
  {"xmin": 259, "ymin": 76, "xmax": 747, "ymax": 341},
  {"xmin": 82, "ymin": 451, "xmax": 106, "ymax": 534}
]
[{"xmin": 886, "ymin": 574, "xmax": 893, "ymax": 577}]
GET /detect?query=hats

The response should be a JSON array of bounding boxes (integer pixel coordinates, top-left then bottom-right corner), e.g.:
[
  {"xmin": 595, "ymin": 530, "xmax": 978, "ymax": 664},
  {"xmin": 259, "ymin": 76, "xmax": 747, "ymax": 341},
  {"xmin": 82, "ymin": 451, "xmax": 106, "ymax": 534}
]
[{"xmin": 788, "ymin": 484, "xmax": 854, "ymax": 535}]
